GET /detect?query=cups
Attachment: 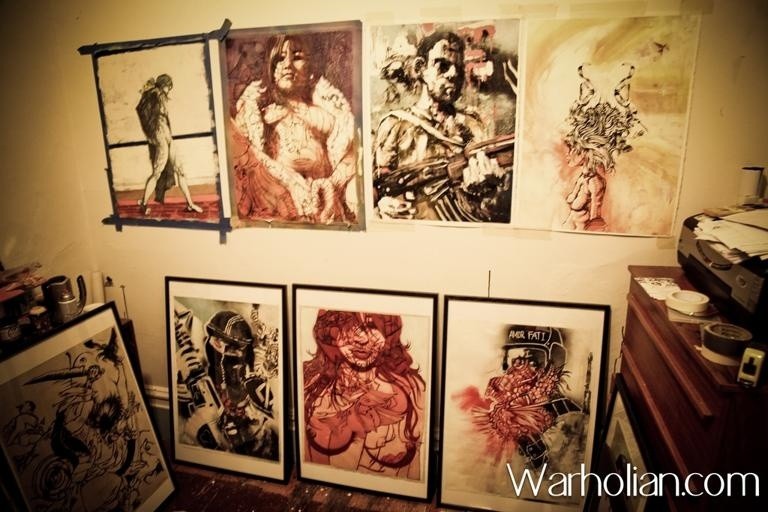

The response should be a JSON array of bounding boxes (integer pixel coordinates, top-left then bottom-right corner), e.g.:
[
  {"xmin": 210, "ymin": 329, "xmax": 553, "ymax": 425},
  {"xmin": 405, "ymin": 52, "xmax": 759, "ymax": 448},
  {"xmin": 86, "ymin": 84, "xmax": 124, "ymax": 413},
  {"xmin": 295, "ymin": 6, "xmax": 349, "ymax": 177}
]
[
  {"xmin": 0, "ymin": 323, "xmax": 22, "ymax": 342},
  {"xmin": 28, "ymin": 305, "xmax": 52, "ymax": 336}
]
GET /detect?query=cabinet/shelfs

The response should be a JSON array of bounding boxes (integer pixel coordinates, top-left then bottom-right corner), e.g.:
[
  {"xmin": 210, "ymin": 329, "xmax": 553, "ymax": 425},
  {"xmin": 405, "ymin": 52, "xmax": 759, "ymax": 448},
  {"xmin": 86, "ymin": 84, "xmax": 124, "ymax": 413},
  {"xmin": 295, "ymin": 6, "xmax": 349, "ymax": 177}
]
[{"xmin": 603, "ymin": 266, "xmax": 766, "ymax": 511}]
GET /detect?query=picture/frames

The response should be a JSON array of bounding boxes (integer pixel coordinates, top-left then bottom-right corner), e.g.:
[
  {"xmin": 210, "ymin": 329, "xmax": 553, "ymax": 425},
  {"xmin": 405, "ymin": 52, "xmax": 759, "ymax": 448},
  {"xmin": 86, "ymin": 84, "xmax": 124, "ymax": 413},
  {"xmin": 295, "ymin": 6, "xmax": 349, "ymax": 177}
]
[
  {"xmin": 166, "ymin": 276, "xmax": 288, "ymax": 486},
  {"xmin": 2, "ymin": 302, "xmax": 174, "ymax": 511},
  {"xmin": 439, "ymin": 294, "xmax": 610, "ymax": 512},
  {"xmin": 77, "ymin": 19, "xmax": 232, "ymax": 245},
  {"xmin": 219, "ymin": 20, "xmax": 366, "ymax": 232},
  {"xmin": 292, "ymin": 283, "xmax": 439, "ymax": 503}
]
